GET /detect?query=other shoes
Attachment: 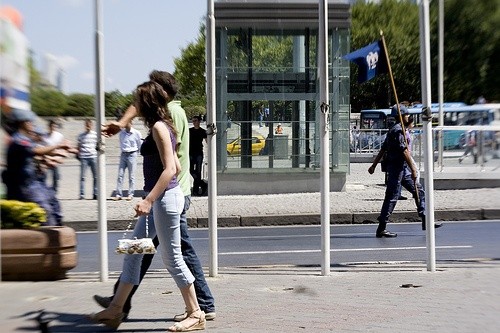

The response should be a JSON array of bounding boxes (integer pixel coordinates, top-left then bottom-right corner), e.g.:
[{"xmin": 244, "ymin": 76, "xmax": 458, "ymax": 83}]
[
  {"xmin": 127, "ymin": 195, "xmax": 133, "ymax": 201},
  {"xmin": 175, "ymin": 310, "xmax": 215, "ymax": 321},
  {"xmin": 192, "ymin": 191, "xmax": 201, "ymax": 196},
  {"xmin": 116, "ymin": 195, "xmax": 121, "ymax": 200},
  {"xmin": 93, "ymin": 295, "xmax": 131, "ymax": 315},
  {"xmin": 201, "ymin": 183, "xmax": 208, "ymax": 194}
]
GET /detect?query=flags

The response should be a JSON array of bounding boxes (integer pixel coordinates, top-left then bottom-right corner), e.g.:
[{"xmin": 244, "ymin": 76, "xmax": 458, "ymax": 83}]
[{"xmin": 339, "ymin": 40, "xmax": 389, "ymax": 86}]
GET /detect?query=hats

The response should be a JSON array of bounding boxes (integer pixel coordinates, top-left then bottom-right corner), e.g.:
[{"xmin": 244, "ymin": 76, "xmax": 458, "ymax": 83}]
[
  {"xmin": 12, "ymin": 108, "xmax": 35, "ymax": 122},
  {"xmin": 31, "ymin": 122, "xmax": 49, "ymax": 136}
]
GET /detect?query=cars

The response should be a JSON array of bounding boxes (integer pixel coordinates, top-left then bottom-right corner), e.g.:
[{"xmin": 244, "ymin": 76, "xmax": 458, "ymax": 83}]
[{"xmin": 225, "ymin": 135, "xmax": 265, "ymax": 157}]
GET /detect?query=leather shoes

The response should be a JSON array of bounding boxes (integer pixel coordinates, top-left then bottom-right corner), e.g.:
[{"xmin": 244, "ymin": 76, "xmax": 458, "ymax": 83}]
[
  {"xmin": 422, "ymin": 215, "xmax": 443, "ymax": 229},
  {"xmin": 376, "ymin": 229, "xmax": 397, "ymax": 238}
]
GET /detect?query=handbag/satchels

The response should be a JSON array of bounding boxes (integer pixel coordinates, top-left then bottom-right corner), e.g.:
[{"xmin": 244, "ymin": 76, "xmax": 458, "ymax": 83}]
[{"xmin": 118, "ymin": 214, "xmax": 156, "ymax": 255}]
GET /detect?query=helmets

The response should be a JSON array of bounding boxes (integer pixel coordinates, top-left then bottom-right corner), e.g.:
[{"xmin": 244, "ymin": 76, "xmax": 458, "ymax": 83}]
[{"xmin": 392, "ymin": 104, "xmax": 409, "ymax": 115}]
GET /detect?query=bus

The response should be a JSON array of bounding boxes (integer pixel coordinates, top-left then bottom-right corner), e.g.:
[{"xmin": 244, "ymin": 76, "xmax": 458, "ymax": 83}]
[{"xmin": 355, "ymin": 103, "xmax": 500, "ymax": 154}]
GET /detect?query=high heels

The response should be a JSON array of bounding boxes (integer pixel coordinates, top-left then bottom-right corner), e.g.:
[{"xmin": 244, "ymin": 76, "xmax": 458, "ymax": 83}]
[
  {"xmin": 85, "ymin": 312, "xmax": 125, "ymax": 329},
  {"xmin": 168, "ymin": 312, "xmax": 207, "ymax": 332}
]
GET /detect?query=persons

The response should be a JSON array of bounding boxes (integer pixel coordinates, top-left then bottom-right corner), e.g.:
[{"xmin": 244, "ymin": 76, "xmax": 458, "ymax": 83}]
[
  {"xmin": 4, "ymin": 71, "xmax": 283, "ymax": 333},
  {"xmin": 368, "ymin": 104, "xmax": 500, "ymax": 238}
]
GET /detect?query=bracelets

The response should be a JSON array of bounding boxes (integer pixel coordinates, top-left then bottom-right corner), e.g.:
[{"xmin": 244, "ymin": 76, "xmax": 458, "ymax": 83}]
[{"xmin": 372, "ymin": 165, "xmax": 375, "ymax": 167}]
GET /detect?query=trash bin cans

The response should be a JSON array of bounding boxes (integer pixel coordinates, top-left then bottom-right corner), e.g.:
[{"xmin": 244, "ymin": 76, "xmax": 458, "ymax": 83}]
[{"xmin": 274, "ymin": 134, "xmax": 289, "ymax": 159}]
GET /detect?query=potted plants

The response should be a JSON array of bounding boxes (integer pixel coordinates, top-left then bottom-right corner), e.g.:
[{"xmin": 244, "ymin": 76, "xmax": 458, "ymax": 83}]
[{"xmin": 0, "ymin": 198, "xmax": 79, "ymax": 280}]
